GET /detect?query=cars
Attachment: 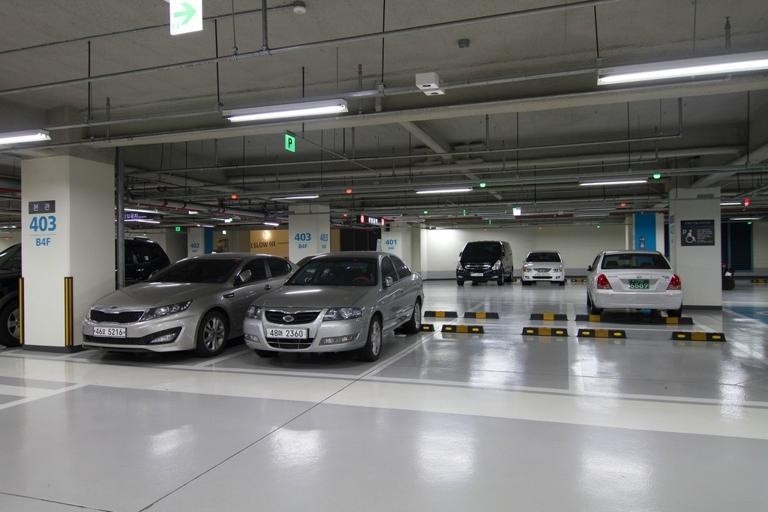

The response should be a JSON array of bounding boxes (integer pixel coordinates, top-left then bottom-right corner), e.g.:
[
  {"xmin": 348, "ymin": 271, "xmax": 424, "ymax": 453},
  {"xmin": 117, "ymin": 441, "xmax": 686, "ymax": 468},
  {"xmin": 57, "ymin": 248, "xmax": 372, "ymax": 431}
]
[
  {"xmin": 83, "ymin": 251, "xmax": 304, "ymax": 357},
  {"xmin": 244, "ymin": 249, "xmax": 423, "ymax": 362},
  {"xmin": 521, "ymin": 250, "xmax": 565, "ymax": 286},
  {"xmin": 587, "ymin": 249, "xmax": 683, "ymax": 318}
]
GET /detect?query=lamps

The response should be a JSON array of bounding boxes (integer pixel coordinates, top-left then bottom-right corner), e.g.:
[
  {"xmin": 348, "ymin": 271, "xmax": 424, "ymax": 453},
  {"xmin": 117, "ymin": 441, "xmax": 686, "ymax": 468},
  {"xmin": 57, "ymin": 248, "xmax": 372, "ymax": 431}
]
[
  {"xmin": 595, "ymin": 50, "xmax": 768, "ymax": 86},
  {"xmin": 0, "ymin": 126, "xmax": 52, "ymax": 147},
  {"xmin": 222, "ymin": 98, "xmax": 350, "ymax": 125}
]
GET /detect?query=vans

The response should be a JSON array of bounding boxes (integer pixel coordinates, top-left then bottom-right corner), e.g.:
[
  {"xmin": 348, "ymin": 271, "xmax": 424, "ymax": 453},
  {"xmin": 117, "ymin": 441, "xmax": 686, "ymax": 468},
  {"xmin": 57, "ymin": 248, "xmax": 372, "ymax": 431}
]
[
  {"xmin": 456, "ymin": 241, "xmax": 513, "ymax": 285},
  {"xmin": 1, "ymin": 238, "xmax": 170, "ymax": 346}
]
